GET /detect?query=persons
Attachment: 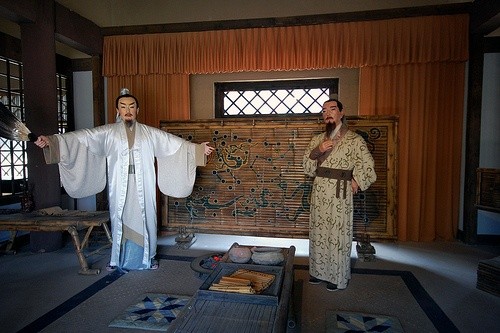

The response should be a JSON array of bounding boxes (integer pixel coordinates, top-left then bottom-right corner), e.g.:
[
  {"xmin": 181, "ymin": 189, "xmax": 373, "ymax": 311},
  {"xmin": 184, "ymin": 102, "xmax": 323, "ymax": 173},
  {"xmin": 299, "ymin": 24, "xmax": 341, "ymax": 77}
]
[
  {"xmin": 34, "ymin": 88, "xmax": 215, "ymax": 270},
  {"xmin": 303, "ymin": 92, "xmax": 378, "ymax": 293}
]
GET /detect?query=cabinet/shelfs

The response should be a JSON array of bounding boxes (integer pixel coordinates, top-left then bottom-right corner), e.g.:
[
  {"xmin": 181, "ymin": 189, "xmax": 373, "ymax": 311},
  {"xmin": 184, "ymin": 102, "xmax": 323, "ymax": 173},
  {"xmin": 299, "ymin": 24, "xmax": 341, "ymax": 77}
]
[{"xmin": 476, "ymin": 257, "xmax": 500, "ymax": 297}]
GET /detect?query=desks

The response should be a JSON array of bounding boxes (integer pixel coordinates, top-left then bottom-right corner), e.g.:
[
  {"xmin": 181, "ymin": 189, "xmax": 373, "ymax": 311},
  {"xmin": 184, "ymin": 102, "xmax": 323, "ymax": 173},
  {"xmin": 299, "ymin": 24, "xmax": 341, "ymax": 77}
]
[
  {"xmin": 0, "ymin": 212, "xmax": 112, "ymax": 275},
  {"xmin": 166, "ymin": 242, "xmax": 296, "ymax": 333}
]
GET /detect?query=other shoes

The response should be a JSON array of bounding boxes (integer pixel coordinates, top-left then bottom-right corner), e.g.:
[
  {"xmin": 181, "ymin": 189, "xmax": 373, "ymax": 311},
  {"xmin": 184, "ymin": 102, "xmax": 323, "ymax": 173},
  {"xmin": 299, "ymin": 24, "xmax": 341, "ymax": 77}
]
[
  {"xmin": 326, "ymin": 282, "xmax": 339, "ymax": 292},
  {"xmin": 150, "ymin": 259, "xmax": 159, "ymax": 269},
  {"xmin": 105, "ymin": 262, "xmax": 117, "ymax": 272},
  {"xmin": 308, "ymin": 277, "xmax": 322, "ymax": 285}
]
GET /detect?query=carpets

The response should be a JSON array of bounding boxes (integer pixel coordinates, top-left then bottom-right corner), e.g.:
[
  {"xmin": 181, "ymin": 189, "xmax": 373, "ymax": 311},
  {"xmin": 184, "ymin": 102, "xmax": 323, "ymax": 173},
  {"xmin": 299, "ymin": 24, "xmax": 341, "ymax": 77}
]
[{"xmin": 18, "ymin": 253, "xmax": 462, "ymax": 333}]
